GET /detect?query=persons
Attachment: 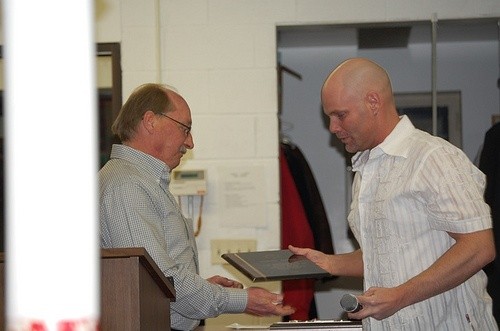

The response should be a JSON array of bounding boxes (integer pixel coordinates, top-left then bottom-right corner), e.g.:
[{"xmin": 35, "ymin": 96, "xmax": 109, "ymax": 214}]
[
  {"xmin": 96, "ymin": 83, "xmax": 296, "ymax": 331},
  {"xmin": 476, "ymin": 75, "xmax": 500, "ymax": 331},
  {"xmin": 277, "ymin": 141, "xmax": 316, "ymax": 325},
  {"xmin": 281, "ymin": 140, "xmax": 339, "ymax": 323},
  {"xmin": 285, "ymin": 56, "xmax": 500, "ymax": 331}
]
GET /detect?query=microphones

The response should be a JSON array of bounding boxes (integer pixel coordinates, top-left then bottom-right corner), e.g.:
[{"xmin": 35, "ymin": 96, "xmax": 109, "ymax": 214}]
[{"xmin": 339, "ymin": 293, "xmax": 363, "ymax": 315}]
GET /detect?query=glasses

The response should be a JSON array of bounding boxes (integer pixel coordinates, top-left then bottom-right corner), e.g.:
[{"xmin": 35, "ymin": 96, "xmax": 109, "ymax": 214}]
[{"xmin": 141, "ymin": 112, "xmax": 191, "ymax": 137}]
